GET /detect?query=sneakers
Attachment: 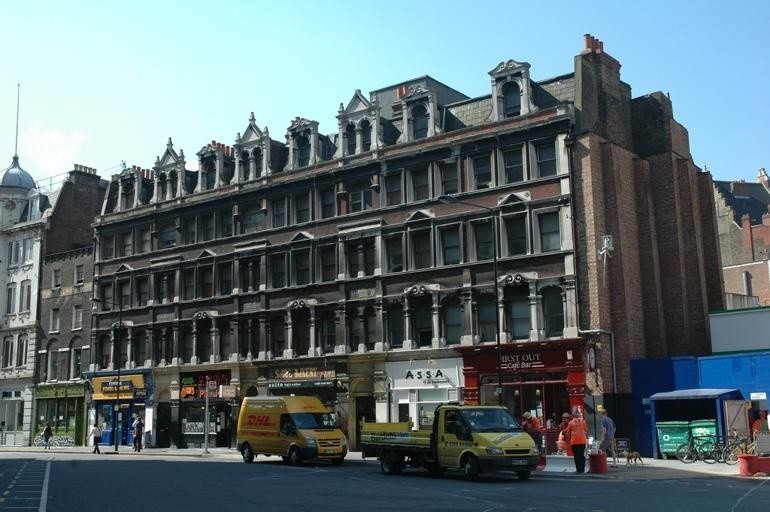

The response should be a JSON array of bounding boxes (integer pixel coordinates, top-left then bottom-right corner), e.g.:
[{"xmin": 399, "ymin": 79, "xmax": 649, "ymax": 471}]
[{"xmin": 577, "ymin": 469, "xmax": 585, "ymax": 474}]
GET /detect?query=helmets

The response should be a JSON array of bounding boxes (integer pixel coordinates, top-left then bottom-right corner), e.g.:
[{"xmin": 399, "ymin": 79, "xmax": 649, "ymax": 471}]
[{"xmin": 523, "ymin": 412, "xmax": 532, "ymax": 420}]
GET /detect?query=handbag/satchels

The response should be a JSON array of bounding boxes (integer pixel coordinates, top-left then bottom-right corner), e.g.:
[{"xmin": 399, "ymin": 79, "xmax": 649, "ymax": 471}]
[
  {"xmin": 99, "ymin": 437, "xmax": 102, "ymax": 443},
  {"xmin": 566, "ymin": 445, "xmax": 575, "ymax": 456}
]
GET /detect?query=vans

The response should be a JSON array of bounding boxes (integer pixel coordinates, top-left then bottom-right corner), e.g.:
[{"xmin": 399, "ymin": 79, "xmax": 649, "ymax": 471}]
[{"xmin": 224, "ymin": 392, "xmax": 349, "ymax": 467}]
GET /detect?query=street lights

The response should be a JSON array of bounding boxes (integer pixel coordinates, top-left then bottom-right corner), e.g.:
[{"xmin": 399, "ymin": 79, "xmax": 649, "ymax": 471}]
[
  {"xmin": 88, "ymin": 296, "xmax": 122, "ymax": 452},
  {"xmin": 438, "ymin": 195, "xmax": 503, "ymax": 409}
]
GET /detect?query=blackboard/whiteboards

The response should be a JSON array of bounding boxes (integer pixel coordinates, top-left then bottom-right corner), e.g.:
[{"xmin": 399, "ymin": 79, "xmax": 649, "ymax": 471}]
[{"xmin": 613, "ymin": 438, "xmax": 631, "ymax": 458}]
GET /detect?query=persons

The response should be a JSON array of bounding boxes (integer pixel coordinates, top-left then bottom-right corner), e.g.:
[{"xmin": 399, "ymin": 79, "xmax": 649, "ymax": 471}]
[
  {"xmin": 132, "ymin": 415, "xmax": 145, "ymax": 452},
  {"xmin": 598, "ymin": 408, "xmax": 618, "ymax": 468},
  {"xmin": 87, "ymin": 423, "xmax": 102, "ymax": 455},
  {"xmin": 559, "ymin": 409, "xmax": 588, "ymax": 474},
  {"xmin": 41, "ymin": 422, "xmax": 53, "ymax": 450},
  {"xmin": 753, "ymin": 410, "xmax": 768, "ymax": 434},
  {"xmin": 522, "ymin": 411, "xmax": 542, "ymax": 452}
]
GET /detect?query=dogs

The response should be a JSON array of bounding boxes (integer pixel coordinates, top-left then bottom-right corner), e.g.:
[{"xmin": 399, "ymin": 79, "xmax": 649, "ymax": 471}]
[{"xmin": 622, "ymin": 449, "xmax": 644, "ymax": 468}]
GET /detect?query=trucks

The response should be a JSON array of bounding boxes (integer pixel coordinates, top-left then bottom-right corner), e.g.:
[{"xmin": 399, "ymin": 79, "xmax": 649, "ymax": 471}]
[{"xmin": 359, "ymin": 399, "xmax": 540, "ymax": 482}]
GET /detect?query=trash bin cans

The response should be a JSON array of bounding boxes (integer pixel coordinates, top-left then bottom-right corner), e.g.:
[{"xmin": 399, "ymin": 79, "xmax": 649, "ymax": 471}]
[{"xmin": 655, "ymin": 419, "xmax": 721, "ymax": 460}]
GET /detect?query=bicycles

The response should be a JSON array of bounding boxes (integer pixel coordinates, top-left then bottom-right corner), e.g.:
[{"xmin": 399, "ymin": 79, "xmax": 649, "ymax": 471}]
[{"xmin": 676, "ymin": 427, "xmax": 756, "ymax": 466}]
